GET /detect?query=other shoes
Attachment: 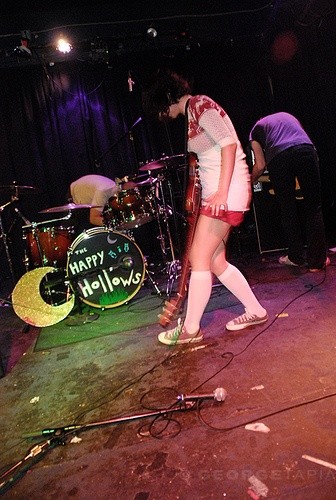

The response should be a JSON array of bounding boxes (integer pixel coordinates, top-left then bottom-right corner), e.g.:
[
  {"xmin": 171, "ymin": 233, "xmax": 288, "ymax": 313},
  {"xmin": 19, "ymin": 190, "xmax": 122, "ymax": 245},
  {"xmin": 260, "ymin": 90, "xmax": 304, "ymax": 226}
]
[
  {"xmin": 309, "ymin": 257, "xmax": 330, "ymax": 272},
  {"xmin": 226, "ymin": 310, "xmax": 269, "ymax": 330},
  {"xmin": 279, "ymin": 256, "xmax": 302, "ymax": 267},
  {"xmin": 158, "ymin": 317, "xmax": 203, "ymax": 345}
]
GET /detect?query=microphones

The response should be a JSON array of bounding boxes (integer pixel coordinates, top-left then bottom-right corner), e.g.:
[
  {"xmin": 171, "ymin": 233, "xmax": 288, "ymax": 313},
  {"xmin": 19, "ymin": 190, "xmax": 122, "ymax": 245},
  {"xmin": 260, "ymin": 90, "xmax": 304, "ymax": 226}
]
[
  {"xmin": 15, "ymin": 208, "xmax": 31, "ymax": 225},
  {"xmin": 128, "ymin": 72, "xmax": 133, "ymax": 92},
  {"xmin": 95, "ymin": 160, "xmax": 100, "ymax": 168},
  {"xmin": 176, "ymin": 387, "xmax": 227, "ymax": 402}
]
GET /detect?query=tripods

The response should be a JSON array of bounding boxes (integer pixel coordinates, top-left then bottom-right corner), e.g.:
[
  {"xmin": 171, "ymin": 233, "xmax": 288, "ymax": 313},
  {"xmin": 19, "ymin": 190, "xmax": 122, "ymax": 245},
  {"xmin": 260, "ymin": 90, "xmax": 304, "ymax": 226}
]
[{"xmin": 156, "ymin": 168, "xmax": 182, "ymax": 296}]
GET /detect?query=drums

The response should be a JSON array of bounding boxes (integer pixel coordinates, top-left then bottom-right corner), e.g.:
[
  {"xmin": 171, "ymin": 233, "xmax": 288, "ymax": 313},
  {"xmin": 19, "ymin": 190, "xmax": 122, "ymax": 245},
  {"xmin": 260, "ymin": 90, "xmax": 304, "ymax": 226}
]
[
  {"xmin": 66, "ymin": 227, "xmax": 145, "ymax": 308},
  {"xmin": 100, "ymin": 185, "xmax": 158, "ymax": 228},
  {"xmin": 22, "ymin": 225, "xmax": 74, "ymax": 268}
]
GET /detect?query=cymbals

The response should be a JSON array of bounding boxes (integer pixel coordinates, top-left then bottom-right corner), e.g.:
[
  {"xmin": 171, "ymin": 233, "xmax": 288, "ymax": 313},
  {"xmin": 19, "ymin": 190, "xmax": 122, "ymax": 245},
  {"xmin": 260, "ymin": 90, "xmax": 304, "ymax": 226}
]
[
  {"xmin": 36, "ymin": 203, "xmax": 104, "ymax": 213},
  {"xmin": 138, "ymin": 177, "xmax": 155, "ymax": 184},
  {"xmin": 139, "ymin": 154, "xmax": 186, "ymax": 170},
  {"xmin": 0, "ymin": 185, "xmax": 40, "ymax": 191}
]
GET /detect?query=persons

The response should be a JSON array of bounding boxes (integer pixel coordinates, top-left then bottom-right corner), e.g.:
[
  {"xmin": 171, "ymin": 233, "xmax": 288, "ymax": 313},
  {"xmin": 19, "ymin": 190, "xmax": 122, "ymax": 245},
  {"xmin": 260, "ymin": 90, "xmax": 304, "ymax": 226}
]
[
  {"xmin": 65, "ymin": 174, "xmax": 137, "ymax": 227},
  {"xmin": 250, "ymin": 110, "xmax": 331, "ymax": 272},
  {"xmin": 142, "ymin": 70, "xmax": 270, "ymax": 345}
]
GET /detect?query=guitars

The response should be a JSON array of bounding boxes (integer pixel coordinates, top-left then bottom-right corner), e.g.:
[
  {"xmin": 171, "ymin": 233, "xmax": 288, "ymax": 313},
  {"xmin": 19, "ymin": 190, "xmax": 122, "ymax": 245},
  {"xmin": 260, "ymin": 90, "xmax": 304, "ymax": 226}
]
[{"xmin": 157, "ymin": 155, "xmax": 202, "ymax": 328}]
